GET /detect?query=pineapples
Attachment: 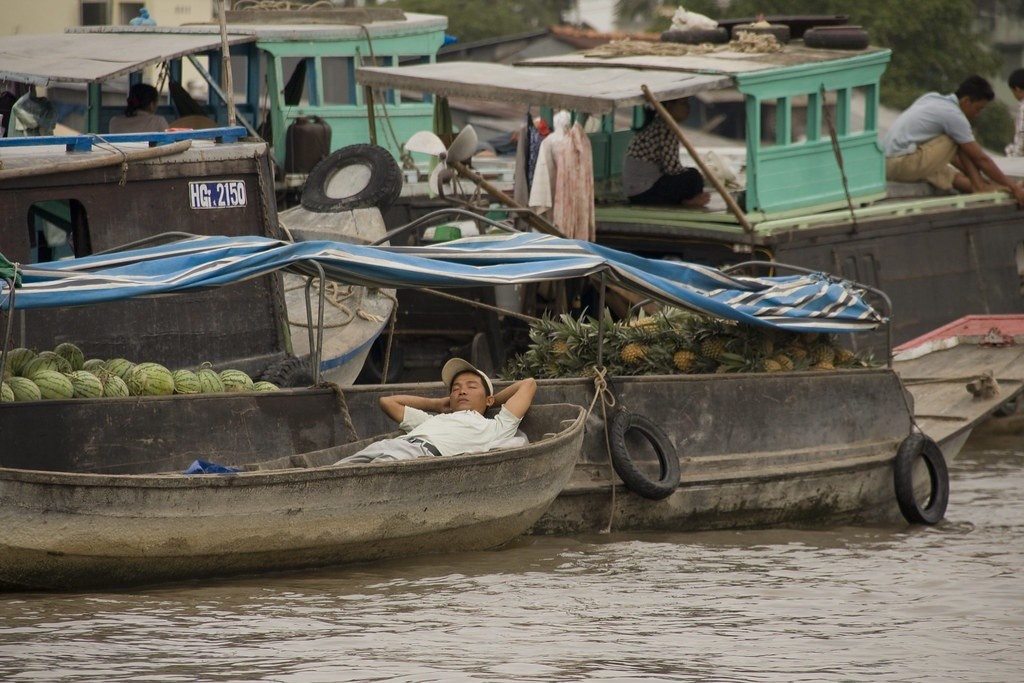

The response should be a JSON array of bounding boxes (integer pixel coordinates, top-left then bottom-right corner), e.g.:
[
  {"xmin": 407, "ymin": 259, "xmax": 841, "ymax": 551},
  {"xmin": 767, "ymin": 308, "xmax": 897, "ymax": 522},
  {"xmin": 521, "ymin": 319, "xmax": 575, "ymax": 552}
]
[{"xmin": 496, "ymin": 304, "xmax": 875, "ymax": 379}]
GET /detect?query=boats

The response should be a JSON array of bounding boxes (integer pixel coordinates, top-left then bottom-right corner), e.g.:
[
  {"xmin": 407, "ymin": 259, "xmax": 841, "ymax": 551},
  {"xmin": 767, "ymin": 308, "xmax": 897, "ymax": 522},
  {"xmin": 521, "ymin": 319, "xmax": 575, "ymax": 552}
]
[
  {"xmin": 0, "ymin": 8, "xmax": 1023, "ymax": 537},
  {"xmin": 0, "ymin": 401, "xmax": 589, "ymax": 592}
]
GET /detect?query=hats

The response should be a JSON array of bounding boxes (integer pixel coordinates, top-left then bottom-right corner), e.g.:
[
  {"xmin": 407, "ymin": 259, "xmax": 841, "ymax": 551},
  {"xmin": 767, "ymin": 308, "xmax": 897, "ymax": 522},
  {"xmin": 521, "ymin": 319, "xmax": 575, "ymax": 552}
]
[{"xmin": 441, "ymin": 357, "xmax": 494, "ymax": 397}]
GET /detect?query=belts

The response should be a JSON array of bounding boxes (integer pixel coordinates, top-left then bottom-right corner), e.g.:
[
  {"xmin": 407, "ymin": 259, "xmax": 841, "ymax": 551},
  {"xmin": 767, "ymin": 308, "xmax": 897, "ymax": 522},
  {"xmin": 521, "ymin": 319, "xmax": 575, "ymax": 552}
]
[{"xmin": 410, "ymin": 437, "xmax": 440, "ymax": 457}]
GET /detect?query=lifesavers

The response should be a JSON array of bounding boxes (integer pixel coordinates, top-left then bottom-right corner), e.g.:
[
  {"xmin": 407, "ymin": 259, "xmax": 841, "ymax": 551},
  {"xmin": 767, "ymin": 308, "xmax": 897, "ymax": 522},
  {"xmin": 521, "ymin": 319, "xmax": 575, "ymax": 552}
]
[
  {"xmin": 610, "ymin": 413, "xmax": 682, "ymax": 500},
  {"xmin": 894, "ymin": 433, "xmax": 951, "ymax": 526},
  {"xmin": 300, "ymin": 142, "xmax": 404, "ymax": 216}
]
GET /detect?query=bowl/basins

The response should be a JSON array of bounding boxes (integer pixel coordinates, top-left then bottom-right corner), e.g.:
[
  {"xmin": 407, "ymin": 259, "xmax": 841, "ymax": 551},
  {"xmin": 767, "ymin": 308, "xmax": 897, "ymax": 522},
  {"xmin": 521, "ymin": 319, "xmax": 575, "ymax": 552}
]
[
  {"xmin": 979, "ymin": 157, "xmax": 1024, "ymax": 185},
  {"xmin": 731, "ymin": 23, "xmax": 790, "ymax": 44},
  {"xmin": 804, "ymin": 26, "xmax": 869, "ymax": 49},
  {"xmin": 660, "ymin": 26, "xmax": 728, "ymax": 45},
  {"xmin": 698, "ymin": 187, "xmax": 746, "ymax": 213}
]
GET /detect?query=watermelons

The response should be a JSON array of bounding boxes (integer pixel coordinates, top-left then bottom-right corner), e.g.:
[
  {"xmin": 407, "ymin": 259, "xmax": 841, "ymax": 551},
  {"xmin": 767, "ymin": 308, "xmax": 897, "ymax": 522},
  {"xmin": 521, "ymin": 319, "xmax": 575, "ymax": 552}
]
[{"xmin": 0, "ymin": 343, "xmax": 283, "ymax": 405}]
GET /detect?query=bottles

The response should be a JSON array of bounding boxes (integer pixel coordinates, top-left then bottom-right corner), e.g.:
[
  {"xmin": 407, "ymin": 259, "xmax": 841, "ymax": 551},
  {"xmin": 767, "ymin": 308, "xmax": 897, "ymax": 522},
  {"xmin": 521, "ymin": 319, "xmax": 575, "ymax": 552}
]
[{"xmin": 626, "ymin": 168, "xmax": 704, "ymax": 207}]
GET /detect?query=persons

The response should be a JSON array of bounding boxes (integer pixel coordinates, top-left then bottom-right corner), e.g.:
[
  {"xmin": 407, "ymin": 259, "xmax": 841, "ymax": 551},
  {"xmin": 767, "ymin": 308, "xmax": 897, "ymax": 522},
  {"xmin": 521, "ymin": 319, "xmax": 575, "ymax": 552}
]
[
  {"xmin": 7, "ymin": 84, "xmax": 56, "ymax": 137},
  {"xmin": 335, "ymin": 357, "xmax": 538, "ymax": 464},
  {"xmin": 108, "ymin": 84, "xmax": 168, "ymax": 134},
  {"xmin": 621, "ymin": 97, "xmax": 712, "ymax": 207},
  {"xmin": 883, "ymin": 75, "xmax": 1024, "ymax": 208},
  {"xmin": 1004, "ymin": 69, "xmax": 1024, "ymax": 158}
]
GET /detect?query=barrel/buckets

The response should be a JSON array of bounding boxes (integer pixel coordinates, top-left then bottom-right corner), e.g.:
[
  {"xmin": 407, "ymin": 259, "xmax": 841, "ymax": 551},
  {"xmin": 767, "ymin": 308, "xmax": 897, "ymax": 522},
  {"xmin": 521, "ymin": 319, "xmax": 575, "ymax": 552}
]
[{"xmin": 284, "ymin": 114, "xmax": 332, "ymax": 173}]
